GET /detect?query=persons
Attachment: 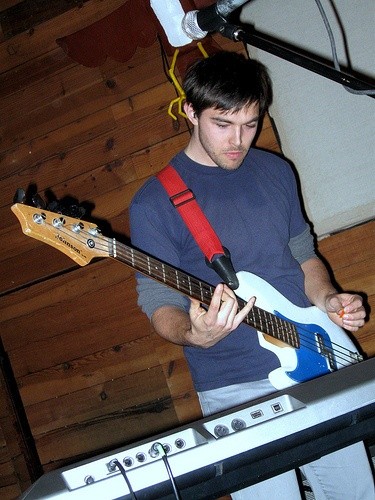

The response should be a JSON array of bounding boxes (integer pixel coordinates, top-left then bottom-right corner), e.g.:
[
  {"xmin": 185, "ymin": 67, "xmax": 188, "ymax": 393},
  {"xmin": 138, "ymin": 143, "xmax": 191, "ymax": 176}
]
[{"xmin": 129, "ymin": 50, "xmax": 375, "ymax": 500}]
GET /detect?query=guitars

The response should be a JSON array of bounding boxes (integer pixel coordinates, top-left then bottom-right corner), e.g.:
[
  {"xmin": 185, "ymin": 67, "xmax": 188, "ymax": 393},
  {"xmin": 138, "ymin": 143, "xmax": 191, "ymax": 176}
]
[{"xmin": 12, "ymin": 192, "xmax": 364, "ymax": 391}]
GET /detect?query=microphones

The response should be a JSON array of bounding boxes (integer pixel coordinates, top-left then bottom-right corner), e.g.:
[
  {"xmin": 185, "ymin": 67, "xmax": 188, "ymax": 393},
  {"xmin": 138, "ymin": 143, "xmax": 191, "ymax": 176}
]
[{"xmin": 181, "ymin": 0, "xmax": 249, "ymax": 41}]
